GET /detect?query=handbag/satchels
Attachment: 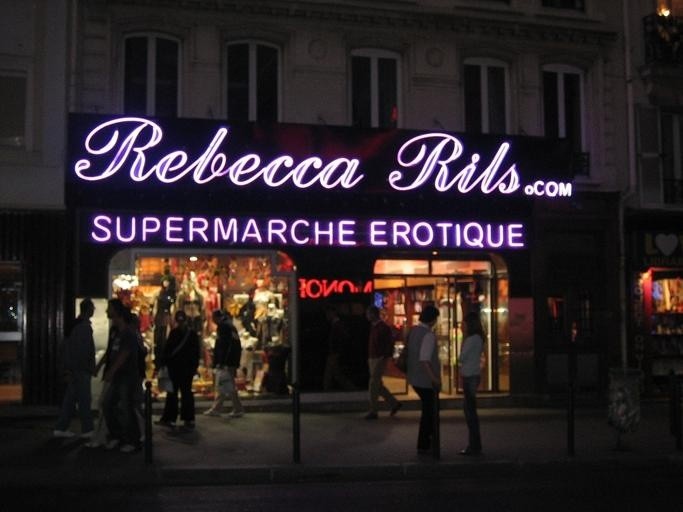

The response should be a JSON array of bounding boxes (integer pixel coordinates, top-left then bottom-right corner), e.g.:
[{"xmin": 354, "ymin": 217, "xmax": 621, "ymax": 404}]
[{"xmin": 395, "ymin": 346, "xmax": 408, "ymax": 370}]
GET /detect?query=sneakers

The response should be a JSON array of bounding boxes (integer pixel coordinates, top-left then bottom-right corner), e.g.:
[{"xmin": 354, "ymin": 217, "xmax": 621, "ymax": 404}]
[
  {"xmin": 155, "ymin": 419, "xmax": 177, "ymax": 426},
  {"xmin": 366, "ymin": 412, "xmax": 378, "ymax": 419},
  {"xmin": 390, "ymin": 403, "xmax": 402, "ymax": 416},
  {"xmin": 81, "ymin": 429, "xmax": 96, "ymax": 438},
  {"xmin": 204, "ymin": 408, "xmax": 219, "ymax": 416},
  {"xmin": 458, "ymin": 449, "xmax": 471, "ymax": 454},
  {"xmin": 121, "ymin": 434, "xmax": 145, "ymax": 452},
  {"xmin": 183, "ymin": 420, "xmax": 195, "ymax": 427},
  {"xmin": 229, "ymin": 410, "xmax": 243, "ymax": 417},
  {"xmin": 54, "ymin": 428, "xmax": 76, "ymax": 438},
  {"xmin": 88, "ymin": 441, "xmax": 100, "ymax": 447}
]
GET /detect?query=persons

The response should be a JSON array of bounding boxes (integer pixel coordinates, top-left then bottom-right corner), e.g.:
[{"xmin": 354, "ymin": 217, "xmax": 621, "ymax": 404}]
[
  {"xmin": 458, "ymin": 312, "xmax": 483, "ymax": 457},
  {"xmin": 110, "ymin": 268, "xmax": 287, "ymax": 394},
  {"xmin": 52, "ymin": 295, "xmax": 240, "ymax": 452},
  {"xmin": 364, "ymin": 304, "xmax": 402, "ymax": 419},
  {"xmin": 405, "ymin": 306, "xmax": 444, "ymax": 461},
  {"xmin": 319, "ymin": 306, "xmax": 361, "ymax": 399}
]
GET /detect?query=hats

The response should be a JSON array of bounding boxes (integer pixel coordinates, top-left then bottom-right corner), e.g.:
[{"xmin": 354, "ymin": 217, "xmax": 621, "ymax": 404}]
[
  {"xmin": 213, "ymin": 310, "xmax": 224, "ymax": 318},
  {"xmin": 175, "ymin": 311, "xmax": 186, "ymax": 320}
]
